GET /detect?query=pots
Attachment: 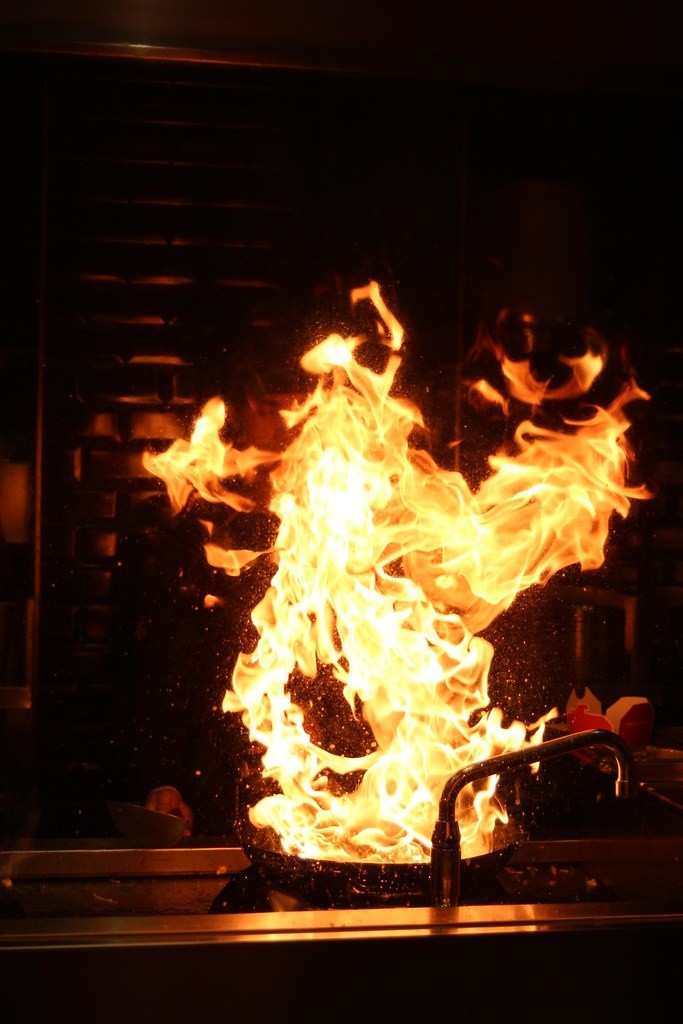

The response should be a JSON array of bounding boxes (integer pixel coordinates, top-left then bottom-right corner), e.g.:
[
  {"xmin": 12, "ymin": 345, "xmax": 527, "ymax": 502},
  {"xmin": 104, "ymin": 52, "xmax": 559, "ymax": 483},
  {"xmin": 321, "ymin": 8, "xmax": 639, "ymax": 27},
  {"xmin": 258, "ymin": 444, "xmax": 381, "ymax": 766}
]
[{"xmin": 241, "ymin": 822, "xmax": 527, "ymax": 912}]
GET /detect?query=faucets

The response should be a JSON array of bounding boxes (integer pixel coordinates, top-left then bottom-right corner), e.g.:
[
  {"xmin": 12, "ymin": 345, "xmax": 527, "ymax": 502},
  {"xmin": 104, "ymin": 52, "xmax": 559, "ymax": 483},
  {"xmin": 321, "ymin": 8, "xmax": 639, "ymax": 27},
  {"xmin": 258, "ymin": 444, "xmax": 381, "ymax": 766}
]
[{"xmin": 428, "ymin": 727, "xmax": 642, "ymax": 903}]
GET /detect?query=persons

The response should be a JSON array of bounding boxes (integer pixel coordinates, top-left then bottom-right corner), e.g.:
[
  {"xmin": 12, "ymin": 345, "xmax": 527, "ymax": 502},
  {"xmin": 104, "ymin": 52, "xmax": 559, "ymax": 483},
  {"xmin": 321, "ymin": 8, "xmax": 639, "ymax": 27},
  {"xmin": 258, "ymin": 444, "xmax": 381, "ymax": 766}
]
[{"xmin": 138, "ymin": 332, "xmax": 332, "ymax": 838}]
